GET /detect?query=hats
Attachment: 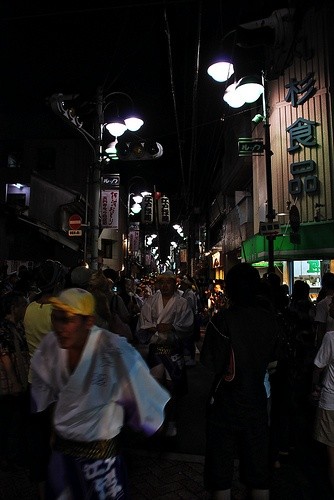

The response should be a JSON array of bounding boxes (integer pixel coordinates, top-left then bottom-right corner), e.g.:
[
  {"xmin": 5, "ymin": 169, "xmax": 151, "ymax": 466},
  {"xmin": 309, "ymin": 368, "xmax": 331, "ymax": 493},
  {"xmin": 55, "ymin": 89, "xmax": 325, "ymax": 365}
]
[
  {"xmin": 48, "ymin": 288, "xmax": 96, "ymax": 317},
  {"xmin": 155, "ymin": 270, "xmax": 177, "ymax": 281}
]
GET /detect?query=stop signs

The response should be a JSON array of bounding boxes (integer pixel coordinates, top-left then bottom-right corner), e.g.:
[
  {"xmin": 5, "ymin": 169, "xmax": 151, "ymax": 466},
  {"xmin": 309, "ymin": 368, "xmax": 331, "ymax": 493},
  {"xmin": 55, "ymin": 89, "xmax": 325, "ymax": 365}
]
[{"xmin": 68, "ymin": 214, "xmax": 83, "ymax": 229}]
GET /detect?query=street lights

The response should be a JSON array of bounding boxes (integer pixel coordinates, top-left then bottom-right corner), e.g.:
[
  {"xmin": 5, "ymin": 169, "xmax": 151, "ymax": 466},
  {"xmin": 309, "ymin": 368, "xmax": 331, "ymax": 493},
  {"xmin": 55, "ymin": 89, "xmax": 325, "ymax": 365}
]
[
  {"xmin": 122, "ymin": 180, "xmax": 153, "ymax": 272},
  {"xmin": 90, "ymin": 88, "xmax": 145, "ymax": 272},
  {"xmin": 208, "ymin": 56, "xmax": 277, "ymax": 277}
]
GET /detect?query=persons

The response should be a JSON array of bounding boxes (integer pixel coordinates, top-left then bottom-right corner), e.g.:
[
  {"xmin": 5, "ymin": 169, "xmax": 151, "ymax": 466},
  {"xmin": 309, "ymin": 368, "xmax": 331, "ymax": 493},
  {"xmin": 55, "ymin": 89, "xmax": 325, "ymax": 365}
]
[
  {"xmin": 0, "ymin": 261, "xmax": 170, "ymax": 500},
  {"xmin": 181, "ymin": 280, "xmax": 196, "ymax": 314},
  {"xmin": 200, "ymin": 263, "xmax": 334, "ymax": 500},
  {"xmin": 139, "ymin": 272, "xmax": 193, "ymax": 408}
]
